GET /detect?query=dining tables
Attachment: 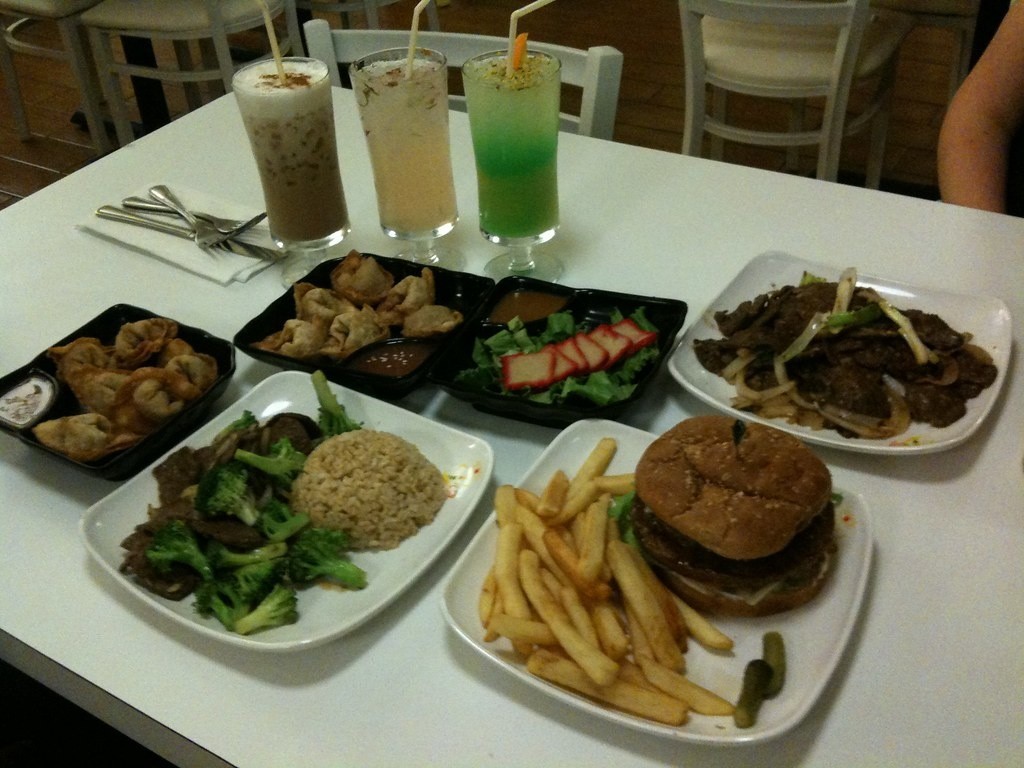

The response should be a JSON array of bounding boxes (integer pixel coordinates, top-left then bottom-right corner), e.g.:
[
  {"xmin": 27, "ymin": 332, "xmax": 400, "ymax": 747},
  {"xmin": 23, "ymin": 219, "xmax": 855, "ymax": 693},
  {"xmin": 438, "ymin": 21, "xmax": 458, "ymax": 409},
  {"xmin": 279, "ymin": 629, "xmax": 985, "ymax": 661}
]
[{"xmin": 0, "ymin": 85, "xmax": 1024, "ymax": 768}]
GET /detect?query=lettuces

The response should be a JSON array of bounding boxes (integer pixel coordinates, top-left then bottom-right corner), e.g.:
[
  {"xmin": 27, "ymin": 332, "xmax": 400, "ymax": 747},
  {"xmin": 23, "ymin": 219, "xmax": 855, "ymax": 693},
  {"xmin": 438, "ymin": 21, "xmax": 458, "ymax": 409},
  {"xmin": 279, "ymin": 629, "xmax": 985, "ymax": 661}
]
[{"xmin": 463, "ymin": 308, "xmax": 660, "ymax": 406}]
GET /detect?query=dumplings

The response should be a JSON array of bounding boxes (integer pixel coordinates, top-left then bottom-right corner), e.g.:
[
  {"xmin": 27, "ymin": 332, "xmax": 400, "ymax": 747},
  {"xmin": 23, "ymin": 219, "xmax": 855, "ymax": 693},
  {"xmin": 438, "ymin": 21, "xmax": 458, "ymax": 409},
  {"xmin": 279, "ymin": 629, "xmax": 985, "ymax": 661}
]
[
  {"xmin": 250, "ymin": 246, "xmax": 464, "ymax": 363},
  {"xmin": 31, "ymin": 317, "xmax": 219, "ymax": 460}
]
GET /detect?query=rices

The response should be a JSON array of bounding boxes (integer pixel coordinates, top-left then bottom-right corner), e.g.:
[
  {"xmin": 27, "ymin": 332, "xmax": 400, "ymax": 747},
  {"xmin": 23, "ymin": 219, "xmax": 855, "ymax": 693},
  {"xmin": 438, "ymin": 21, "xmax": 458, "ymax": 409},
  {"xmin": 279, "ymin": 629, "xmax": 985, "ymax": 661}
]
[{"xmin": 290, "ymin": 427, "xmax": 448, "ymax": 551}]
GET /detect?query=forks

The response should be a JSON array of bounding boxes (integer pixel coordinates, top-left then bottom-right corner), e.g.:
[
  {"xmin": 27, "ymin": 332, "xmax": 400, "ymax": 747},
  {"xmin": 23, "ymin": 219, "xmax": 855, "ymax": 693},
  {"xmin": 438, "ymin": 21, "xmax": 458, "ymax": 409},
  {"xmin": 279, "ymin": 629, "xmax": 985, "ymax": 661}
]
[
  {"xmin": 121, "ymin": 196, "xmax": 267, "ymax": 235},
  {"xmin": 151, "ymin": 184, "xmax": 234, "ymax": 250}
]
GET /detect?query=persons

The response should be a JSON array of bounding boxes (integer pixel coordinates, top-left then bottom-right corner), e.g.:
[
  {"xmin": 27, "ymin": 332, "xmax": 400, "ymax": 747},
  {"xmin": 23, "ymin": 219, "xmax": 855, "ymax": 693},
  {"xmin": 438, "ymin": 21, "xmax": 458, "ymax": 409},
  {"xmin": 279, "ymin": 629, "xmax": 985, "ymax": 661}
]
[{"xmin": 938, "ymin": 0, "xmax": 1024, "ymax": 214}]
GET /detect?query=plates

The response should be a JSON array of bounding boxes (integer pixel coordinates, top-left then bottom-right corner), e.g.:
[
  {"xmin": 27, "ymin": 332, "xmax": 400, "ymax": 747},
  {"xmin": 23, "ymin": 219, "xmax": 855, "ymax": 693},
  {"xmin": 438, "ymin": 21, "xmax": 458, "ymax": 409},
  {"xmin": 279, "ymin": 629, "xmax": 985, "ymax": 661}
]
[
  {"xmin": 425, "ymin": 274, "xmax": 688, "ymax": 431},
  {"xmin": 76, "ymin": 370, "xmax": 496, "ymax": 653},
  {"xmin": 441, "ymin": 420, "xmax": 873, "ymax": 746},
  {"xmin": 668, "ymin": 249, "xmax": 1012, "ymax": 456},
  {"xmin": 233, "ymin": 252, "xmax": 495, "ymax": 394},
  {"xmin": 0, "ymin": 303, "xmax": 236, "ymax": 480}
]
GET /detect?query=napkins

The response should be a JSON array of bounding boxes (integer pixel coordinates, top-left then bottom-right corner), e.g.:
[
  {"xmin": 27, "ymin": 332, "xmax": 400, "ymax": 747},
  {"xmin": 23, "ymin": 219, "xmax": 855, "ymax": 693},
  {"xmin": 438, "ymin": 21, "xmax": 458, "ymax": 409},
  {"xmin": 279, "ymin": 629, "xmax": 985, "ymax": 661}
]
[{"xmin": 76, "ymin": 179, "xmax": 287, "ymax": 284}]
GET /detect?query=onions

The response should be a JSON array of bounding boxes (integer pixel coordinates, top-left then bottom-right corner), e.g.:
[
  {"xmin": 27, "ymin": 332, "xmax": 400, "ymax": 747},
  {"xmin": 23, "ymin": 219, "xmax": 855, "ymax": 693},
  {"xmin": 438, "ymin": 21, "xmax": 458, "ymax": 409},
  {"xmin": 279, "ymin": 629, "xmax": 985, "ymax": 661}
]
[{"xmin": 722, "ymin": 267, "xmax": 930, "ymax": 440}]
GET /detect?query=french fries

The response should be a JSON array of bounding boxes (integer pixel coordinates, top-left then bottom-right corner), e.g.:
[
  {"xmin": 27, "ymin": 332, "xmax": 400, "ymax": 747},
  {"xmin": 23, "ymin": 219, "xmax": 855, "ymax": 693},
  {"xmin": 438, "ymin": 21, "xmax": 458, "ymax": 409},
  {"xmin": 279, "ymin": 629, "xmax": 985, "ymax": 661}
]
[{"xmin": 477, "ymin": 437, "xmax": 784, "ymax": 729}]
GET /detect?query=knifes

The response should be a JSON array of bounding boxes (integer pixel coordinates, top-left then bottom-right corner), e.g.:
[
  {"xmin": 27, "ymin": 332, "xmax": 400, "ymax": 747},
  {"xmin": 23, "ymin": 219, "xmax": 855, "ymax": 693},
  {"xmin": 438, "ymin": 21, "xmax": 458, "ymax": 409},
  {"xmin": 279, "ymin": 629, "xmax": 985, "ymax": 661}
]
[{"xmin": 96, "ymin": 205, "xmax": 288, "ymax": 262}]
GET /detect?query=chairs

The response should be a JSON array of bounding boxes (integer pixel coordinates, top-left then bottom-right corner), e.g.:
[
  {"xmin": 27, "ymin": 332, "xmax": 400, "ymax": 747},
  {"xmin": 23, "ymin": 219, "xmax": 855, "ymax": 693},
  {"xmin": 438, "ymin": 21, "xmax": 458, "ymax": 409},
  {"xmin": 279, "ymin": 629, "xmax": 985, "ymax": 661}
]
[
  {"xmin": 302, "ymin": 18, "xmax": 625, "ymax": 141},
  {"xmin": 680, "ymin": 0, "xmax": 912, "ymax": 192},
  {"xmin": 0, "ymin": 0, "xmax": 114, "ymax": 159},
  {"xmin": 81, "ymin": 0, "xmax": 306, "ymax": 149}
]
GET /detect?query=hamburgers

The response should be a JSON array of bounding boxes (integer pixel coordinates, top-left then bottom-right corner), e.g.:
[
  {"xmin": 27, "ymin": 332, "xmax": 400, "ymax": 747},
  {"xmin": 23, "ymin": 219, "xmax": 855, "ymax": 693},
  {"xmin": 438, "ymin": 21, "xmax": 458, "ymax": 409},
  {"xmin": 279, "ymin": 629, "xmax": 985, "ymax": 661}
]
[{"xmin": 604, "ymin": 415, "xmax": 836, "ymax": 616}]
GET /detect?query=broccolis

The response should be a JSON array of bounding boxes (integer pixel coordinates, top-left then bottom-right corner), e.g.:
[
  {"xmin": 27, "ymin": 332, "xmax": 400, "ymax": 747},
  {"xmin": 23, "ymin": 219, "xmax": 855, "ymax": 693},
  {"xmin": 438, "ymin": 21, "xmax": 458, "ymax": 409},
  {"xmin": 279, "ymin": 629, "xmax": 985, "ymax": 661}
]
[{"xmin": 139, "ymin": 372, "xmax": 369, "ymax": 636}]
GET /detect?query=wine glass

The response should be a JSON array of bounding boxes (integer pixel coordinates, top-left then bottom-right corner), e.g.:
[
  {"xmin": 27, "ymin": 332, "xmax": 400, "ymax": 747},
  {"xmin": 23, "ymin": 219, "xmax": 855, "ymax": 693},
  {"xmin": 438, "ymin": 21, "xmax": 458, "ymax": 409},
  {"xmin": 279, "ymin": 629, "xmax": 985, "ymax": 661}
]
[
  {"xmin": 461, "ymin": 49, "xmax": 565, "ymax": 285},
  {"xmin": 349, "ymin": 47, "xmax": 467, "ymax": 272},
  {"xmin": 231, "ymin": 56, "xmax": 351, "ymax": 286}
]
